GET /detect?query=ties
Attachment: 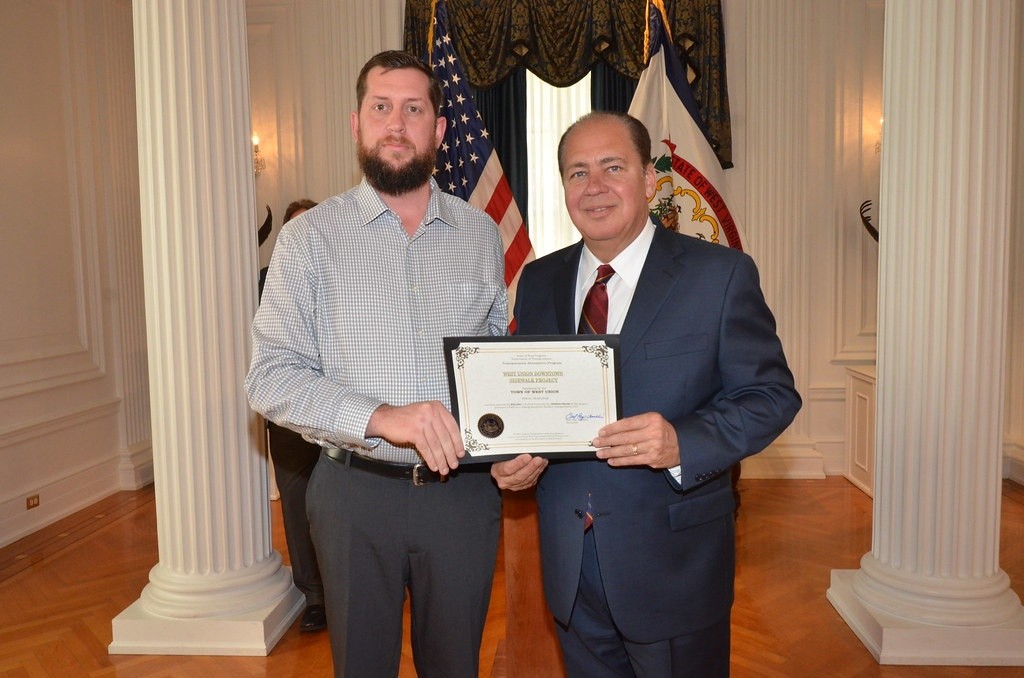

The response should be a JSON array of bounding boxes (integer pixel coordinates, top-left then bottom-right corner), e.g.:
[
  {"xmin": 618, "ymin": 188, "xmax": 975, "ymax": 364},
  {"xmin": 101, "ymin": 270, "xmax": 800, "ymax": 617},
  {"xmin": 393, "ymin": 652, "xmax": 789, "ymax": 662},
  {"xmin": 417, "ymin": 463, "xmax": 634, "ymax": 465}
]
[{"xmin": 577, "ymin": 265, "xmax": 616, "ymax": 335}]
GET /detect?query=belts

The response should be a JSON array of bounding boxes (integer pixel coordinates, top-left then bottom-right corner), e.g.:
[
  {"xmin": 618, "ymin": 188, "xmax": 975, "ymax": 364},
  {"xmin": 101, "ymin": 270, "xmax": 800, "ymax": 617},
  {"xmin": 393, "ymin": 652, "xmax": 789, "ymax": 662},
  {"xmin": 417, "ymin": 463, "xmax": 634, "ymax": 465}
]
[{"xmin": 322, "ymin": 445, "xmax": 449, "ymax": 483}]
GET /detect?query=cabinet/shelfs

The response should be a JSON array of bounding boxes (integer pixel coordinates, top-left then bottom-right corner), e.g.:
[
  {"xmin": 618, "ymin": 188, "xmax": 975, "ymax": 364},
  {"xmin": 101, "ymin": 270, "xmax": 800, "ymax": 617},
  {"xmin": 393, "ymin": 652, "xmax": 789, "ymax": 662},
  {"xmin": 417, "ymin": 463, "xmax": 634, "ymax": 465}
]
[{"xmin": 844, "ymin": 364, "xmax": 875, "ymax": 499}]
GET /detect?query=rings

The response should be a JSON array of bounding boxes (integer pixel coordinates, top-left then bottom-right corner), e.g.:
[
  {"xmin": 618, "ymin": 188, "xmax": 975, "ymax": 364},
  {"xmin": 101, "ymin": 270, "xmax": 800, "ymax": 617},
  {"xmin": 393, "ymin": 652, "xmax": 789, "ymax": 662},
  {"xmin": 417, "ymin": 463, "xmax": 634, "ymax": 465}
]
[{"xmin": 631, "ymin": 443, "xmax": 640, "ymax": 456}]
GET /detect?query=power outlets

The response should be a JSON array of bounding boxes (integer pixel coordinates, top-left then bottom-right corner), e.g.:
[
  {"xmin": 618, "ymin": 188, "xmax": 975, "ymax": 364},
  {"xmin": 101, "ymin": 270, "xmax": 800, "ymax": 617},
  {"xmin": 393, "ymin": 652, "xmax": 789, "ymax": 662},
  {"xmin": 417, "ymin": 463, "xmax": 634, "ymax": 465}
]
[{"xmin": 27, "ymin": 494, "xmax": 39, "ymax": 510}]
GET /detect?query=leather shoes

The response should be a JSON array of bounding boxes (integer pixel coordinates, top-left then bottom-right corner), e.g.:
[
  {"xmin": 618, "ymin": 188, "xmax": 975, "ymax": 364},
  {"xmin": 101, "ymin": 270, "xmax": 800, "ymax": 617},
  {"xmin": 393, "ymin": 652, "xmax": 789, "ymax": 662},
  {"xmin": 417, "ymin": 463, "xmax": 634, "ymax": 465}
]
[{"xmin": 300, "ymin": 604, "xmax": 326, "ymax": 631}]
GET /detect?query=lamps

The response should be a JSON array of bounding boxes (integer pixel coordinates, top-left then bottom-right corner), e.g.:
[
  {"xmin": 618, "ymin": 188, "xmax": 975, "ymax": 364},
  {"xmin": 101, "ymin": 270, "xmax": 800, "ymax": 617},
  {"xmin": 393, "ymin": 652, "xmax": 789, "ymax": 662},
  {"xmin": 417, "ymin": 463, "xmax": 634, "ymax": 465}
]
[{"xmin": 253, "ymin": 133, "xmax": 266, "ymax": 177}]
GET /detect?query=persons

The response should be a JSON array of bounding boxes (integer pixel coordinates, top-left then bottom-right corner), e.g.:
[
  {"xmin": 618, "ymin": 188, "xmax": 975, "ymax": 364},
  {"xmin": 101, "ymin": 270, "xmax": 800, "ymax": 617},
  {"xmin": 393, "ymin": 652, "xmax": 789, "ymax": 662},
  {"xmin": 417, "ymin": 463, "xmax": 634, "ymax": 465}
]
[
  {"xmin": 243, "ymin": 48, "xmax": 511, "ymax": 678},
  {"xmin": 489, "ymin": 113, "xmax": 803, "ymax": 676},
  {"xmin": 257, "ymin": 196, "xmax": 326, "ymax": 637}
]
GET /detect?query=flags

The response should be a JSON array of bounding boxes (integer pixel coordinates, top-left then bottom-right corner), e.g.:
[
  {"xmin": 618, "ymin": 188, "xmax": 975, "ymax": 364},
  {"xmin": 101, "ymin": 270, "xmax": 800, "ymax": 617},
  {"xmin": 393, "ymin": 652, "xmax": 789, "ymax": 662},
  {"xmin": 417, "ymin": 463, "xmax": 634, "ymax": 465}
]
[
  {"xmin": 625, "ymin": 1, "xmax": 748, "ymax": 257},
  {"xmin": 425, "ymin": 4, "xmax": 536, "ymax": 337}
]
